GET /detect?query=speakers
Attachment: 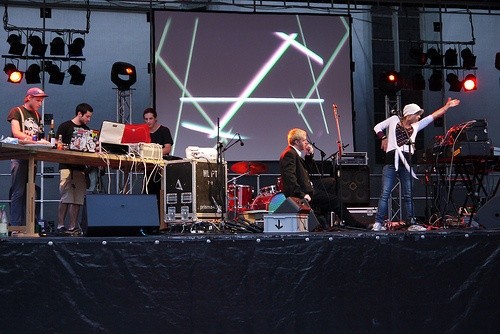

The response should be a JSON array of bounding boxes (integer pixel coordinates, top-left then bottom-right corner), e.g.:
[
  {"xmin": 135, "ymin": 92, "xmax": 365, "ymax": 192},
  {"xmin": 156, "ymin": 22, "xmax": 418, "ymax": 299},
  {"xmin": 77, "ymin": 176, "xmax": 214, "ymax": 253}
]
[
  {"xmin": 331, "ymin": 164, "xmax": 371, "ymax": 206},
  {"xmin": 273, "ymin": 197, "xmax": 320, "ymax": 232},
  {"xmin": 80, "ymin": 194, "xmax": 159, "ymax": 236},
  {"xmin": 475, "ymin": 194, "xmax": 500, "ymax": 229}
]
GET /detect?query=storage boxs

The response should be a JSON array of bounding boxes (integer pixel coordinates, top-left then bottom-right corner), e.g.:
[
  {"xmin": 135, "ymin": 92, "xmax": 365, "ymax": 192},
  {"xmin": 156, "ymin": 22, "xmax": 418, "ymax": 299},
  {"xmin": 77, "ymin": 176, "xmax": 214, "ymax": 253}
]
[
  {"xmin": 166, "ymin": 160, "xmax": 227, "ymax": 222},
  {"xmin": 263, "ymin": 214, "xmax": 309, "ymax": 234}
]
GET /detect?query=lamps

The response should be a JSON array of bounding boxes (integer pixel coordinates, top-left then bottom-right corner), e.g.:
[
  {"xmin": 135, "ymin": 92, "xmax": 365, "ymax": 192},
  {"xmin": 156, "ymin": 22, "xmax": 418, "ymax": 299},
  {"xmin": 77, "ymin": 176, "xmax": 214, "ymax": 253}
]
[
  {"xmin": 1, "ymin": 29, "xmax": 86, "ymax": 86},
  {"xmin": 380, "ymin": 42, "xmax": 478, "ymax": 94}
]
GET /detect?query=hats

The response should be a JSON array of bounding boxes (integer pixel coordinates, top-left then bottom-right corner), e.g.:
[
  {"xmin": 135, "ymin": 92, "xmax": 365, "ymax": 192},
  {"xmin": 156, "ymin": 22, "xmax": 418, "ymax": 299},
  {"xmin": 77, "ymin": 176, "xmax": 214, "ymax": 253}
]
[
  {"xmin": 25, "ymin": 87, "xmax": 48, "ymax": 99},
  {"xmin": 402, "ymin": 103, "xmax": 425, "ymax": 117}
]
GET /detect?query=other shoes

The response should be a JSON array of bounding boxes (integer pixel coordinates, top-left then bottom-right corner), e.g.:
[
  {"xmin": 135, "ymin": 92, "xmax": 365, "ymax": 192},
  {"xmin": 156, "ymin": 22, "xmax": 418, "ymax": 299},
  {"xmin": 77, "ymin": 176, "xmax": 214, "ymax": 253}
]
[
  {"xmin": 317, "ymin": 214, "xmax": 329, "ymax": 230},
  {"xmin": 54, "ymin": 226, "xmax": 81, "ymax": 237},
  {"xmin": 345, "ymin": 223, "xmax": 369, "ymax": 231}
]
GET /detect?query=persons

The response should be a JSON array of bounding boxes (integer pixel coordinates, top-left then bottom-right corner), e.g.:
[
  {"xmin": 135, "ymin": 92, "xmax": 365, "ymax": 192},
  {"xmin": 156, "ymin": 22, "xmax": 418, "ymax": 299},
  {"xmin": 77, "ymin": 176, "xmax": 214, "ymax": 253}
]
[
  {"xmin": 373, "ymin": 97, "xmax": 460, "ymax": 231},
  {"xmin": 55, "ymin": 103, "xmax": 94, "ymax": 233},
  {"xmin": 143, "ymin": 108, "xmax": 173, "ymax": 155},
  {"xmin": 279, "ymin": 128, "xmax": 371, "ymax": 229},
  {"xmin": 7, "ymin": 87, "xmax": 49, "ymax": 237}
]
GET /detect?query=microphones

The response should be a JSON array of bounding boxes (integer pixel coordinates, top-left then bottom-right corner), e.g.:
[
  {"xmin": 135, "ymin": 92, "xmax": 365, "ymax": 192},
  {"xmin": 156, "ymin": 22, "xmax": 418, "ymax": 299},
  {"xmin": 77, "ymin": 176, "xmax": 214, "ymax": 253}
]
[
  {"xmin": 391, "ymin": 110, "xmax": 401, "ymax": 114},
  {"xmin": 238, "ymin": 133, "xmax": 244, "ymax": 146}
]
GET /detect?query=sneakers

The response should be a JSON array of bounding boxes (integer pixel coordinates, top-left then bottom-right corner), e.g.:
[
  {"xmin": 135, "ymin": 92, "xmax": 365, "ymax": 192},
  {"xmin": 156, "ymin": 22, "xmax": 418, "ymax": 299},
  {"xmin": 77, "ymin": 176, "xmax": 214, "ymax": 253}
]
[
  {"xmin": 373, "ymin": 222, "xmax": 382, "ymax": 231},
  {"xmin": 408, "ymin": 225, "xmax": 426, "ymax": 232}
]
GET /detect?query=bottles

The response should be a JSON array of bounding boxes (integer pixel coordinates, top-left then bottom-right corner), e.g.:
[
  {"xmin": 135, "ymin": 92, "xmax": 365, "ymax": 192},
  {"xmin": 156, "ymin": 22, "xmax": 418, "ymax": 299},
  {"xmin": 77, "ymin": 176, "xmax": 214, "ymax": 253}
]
[
  {"xmin": 32, "ymin": 119, "xmax": 62, "ymax": 149},
  {"xmin": 0, "ymin": 205, "xmax": 8, "ymax": 237}
]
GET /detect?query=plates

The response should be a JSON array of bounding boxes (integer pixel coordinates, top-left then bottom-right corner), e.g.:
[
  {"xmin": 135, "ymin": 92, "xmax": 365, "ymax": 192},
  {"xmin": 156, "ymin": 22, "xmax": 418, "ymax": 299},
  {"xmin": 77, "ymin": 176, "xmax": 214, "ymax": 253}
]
[{"xmin": 24, "ymin": 144, "xmax": 54, "ymax": 148}]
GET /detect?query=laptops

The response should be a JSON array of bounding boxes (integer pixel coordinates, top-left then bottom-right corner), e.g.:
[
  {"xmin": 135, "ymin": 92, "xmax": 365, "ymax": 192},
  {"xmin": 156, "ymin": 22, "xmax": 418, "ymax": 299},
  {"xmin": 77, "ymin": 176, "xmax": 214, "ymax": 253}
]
[{"xmin": 98, "ymin": 121, "xmax": 151, "ymax": 144}]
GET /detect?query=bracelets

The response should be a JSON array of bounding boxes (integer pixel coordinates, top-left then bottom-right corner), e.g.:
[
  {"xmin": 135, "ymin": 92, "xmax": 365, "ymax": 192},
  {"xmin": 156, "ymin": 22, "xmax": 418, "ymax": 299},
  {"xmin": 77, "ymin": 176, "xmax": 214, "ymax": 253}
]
[{"xmin": 380, "ymin": 136, "xmax": 386, "ymax": 140}]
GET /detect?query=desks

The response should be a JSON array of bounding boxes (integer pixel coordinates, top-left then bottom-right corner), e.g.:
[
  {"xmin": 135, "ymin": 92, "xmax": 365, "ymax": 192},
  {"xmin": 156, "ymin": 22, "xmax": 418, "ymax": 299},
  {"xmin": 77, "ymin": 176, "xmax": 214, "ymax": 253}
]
[
  {"xmin": 0, "ymin": 139, "xmax": 166, "ymax": 237},
  {"xmin": 422, "ymin": 155, "xmax": 500, "ymax": 229}
]
